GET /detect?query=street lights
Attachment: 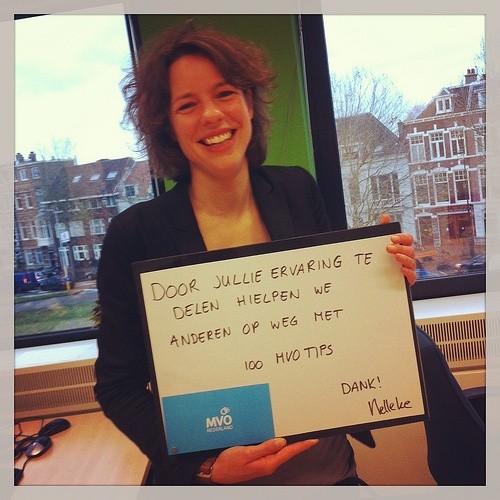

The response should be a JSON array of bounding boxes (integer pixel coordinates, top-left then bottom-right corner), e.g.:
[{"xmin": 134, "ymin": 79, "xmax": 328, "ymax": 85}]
[
  {"xmin": 39, "ymin": 208, "xmax": 71, "ymax": 291},
  {"xmin": 456, "ymin": 147, "xmax": 476, "ymax": 258}
]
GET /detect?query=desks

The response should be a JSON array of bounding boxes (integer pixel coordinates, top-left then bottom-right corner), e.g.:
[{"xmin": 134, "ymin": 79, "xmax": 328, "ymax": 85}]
[{"xmin": 14, "ymin": 408, "xmax": 152, "ymax": 485}]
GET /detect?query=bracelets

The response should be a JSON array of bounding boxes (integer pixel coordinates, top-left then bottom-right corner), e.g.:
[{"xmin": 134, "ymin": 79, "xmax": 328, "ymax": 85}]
[{"xmin": 197, "ymin": 455, "xmax": 218, "ymax": 485}]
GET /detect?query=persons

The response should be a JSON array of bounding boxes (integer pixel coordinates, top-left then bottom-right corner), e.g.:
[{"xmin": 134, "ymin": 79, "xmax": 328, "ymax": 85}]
[{"xmin": 89, "ymin": 20, "xmax": 424, "ymax": 486}]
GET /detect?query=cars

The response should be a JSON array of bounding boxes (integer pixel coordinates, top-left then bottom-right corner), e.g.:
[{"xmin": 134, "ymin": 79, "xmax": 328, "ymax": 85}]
[
  {"xmin": 456, "ymin": 254, "xmax": 485, "ymax": 274},
  {"xmin": 14, "ymin": 262, "xmax": 99, "ymax": 294}
]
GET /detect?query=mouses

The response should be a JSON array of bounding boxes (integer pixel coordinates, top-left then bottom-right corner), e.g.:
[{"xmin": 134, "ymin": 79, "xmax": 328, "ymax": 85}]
[
  {"xmin": 38, "ymin": 418, "xmax": 71, "ymax": 436},
  {"xmin": 24, "ymin": 436, "xmax": 53, "ymax": 458}
]
